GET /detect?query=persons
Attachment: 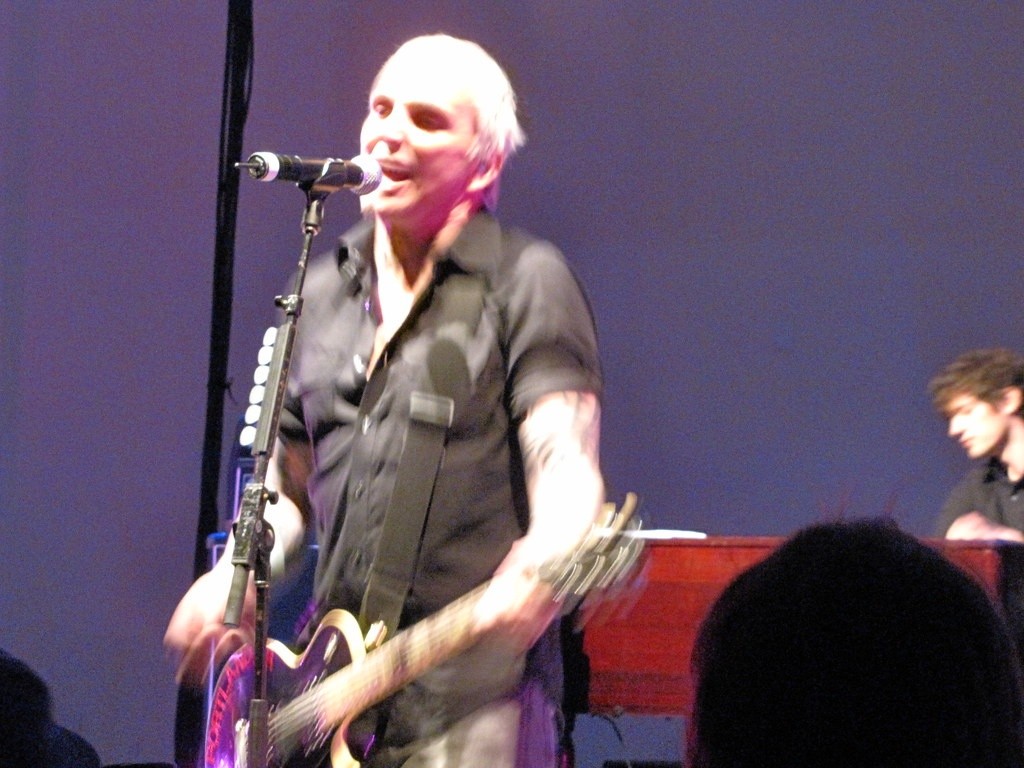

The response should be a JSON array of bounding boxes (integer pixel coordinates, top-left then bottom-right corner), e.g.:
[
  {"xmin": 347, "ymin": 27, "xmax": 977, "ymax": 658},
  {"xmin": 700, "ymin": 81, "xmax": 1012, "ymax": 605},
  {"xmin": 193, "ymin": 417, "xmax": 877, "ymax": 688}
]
[
  {"xmin": 1, "ymin": 646, "xmax": 102, "ymax": 767},
  {"xmin": 164, "ymin": 34, "xmax": 607, "ymax": 768},
  {"xmin": 683, "ymin": 512, "xmax": 1024, "ymax": 768},
  {"xmin": 925, "ymin": 347, "xmax": 1024, "ymax": 541}
]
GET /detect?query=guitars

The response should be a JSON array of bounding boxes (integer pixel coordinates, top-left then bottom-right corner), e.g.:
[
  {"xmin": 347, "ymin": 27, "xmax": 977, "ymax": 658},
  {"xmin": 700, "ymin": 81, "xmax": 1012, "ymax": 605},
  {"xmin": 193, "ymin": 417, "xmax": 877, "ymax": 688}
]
[{"xmin": 202, "ymin": 489, "xmax": 657, "ymax": 768}]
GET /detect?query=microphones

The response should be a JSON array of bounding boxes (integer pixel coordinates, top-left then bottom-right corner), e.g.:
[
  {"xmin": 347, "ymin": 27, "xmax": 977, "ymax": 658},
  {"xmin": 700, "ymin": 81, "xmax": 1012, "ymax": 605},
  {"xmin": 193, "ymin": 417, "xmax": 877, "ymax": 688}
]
[{"xmin": 233, "ymin": 151, "xmax": 383, "ymax": 195}]
